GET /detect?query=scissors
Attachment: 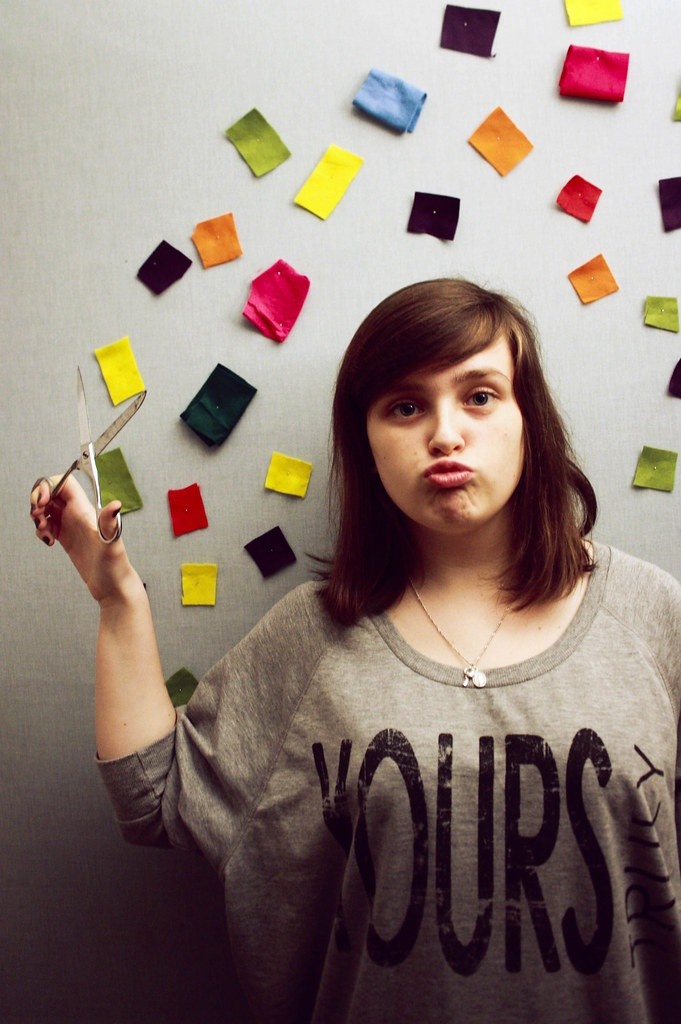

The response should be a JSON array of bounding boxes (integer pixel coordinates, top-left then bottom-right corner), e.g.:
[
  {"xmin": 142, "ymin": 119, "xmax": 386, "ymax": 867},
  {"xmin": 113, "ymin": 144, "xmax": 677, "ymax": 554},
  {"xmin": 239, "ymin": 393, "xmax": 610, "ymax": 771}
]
[{"xmin": 30, "ymin": 364, "xmax": 147, "ymax": 545}]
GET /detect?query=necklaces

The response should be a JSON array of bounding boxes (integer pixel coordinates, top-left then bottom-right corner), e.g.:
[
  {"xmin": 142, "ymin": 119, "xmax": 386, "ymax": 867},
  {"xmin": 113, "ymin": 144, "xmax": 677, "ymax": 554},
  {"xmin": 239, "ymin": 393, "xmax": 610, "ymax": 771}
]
[{"xmin": 409, "ymin": 576, "xmax": 518, "ymax": 689}]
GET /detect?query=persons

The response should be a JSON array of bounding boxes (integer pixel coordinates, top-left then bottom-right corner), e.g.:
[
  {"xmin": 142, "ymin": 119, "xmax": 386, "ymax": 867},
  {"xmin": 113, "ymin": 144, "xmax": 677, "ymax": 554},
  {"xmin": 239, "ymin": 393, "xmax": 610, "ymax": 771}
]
[{"xmin": 29, "ymin": 278, "xmax": 681, "ymax": 1024}]
